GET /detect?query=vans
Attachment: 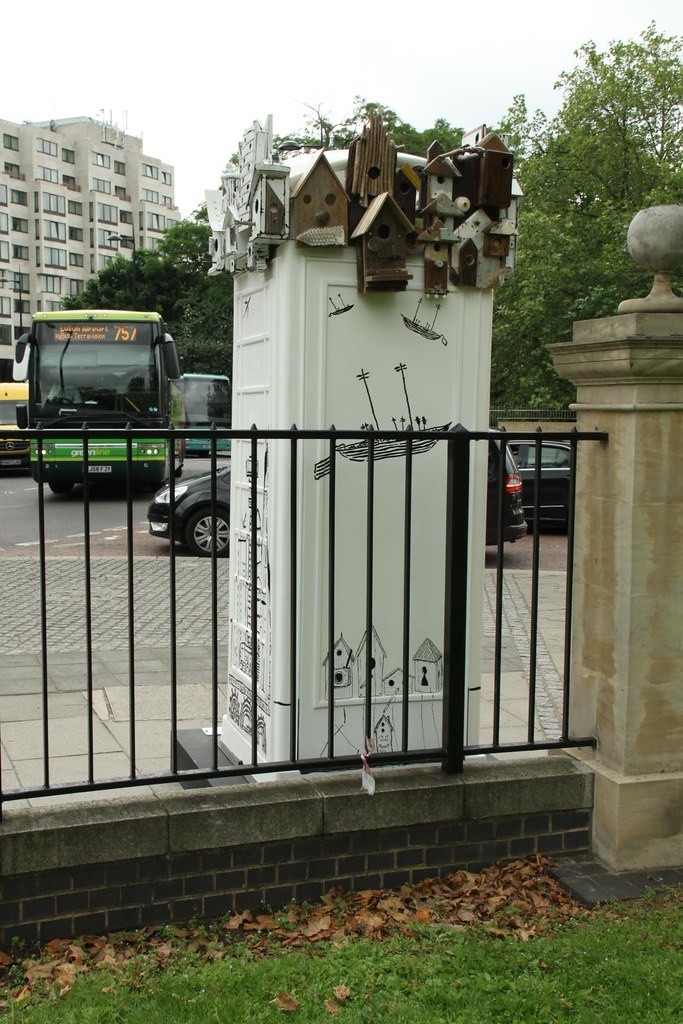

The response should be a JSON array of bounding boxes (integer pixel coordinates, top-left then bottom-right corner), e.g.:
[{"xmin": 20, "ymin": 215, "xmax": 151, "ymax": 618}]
[
  {"xmin": 148, "ymin": 428, "xmax": 526, "ymax": 554},
  {"xmin": 0, "ymin": 382, "xmax": 30, "ymax": 469}
]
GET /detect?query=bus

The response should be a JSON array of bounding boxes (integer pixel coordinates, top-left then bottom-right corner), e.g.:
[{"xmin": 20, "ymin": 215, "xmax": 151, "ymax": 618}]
[
  {"xmin": 182, "ymin": 372, "xmax": 231, "ymax": 456},
  {"xmin": 15, "ymin": 310, "xmax": 185, "ymax": 494}
]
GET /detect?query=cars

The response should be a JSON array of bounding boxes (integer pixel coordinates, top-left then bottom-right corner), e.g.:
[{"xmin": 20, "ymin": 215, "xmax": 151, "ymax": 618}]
[{"xmin": 509, "ymin": 441, "xmax": 572, "ymax": 523}]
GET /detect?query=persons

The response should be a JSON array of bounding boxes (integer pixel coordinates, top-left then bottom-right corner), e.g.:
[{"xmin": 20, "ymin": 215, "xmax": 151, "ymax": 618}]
[{"xmin": 48, "ymin": 371, "xmax": 82, "ymax": 403}]
